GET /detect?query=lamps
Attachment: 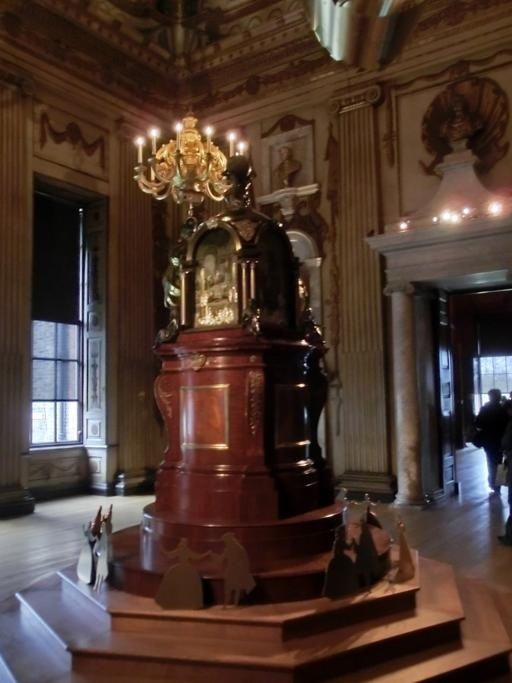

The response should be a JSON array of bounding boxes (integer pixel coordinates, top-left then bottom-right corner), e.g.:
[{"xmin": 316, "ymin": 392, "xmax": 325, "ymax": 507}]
[{"xmin": 133, "ymin": 102, "xmax": 249, "ymax": 217}]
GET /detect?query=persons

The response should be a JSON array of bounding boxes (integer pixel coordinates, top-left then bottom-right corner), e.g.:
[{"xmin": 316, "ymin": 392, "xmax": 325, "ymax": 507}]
[
  {"xmin": 271, "ymin": 144, "xmax": 302, "ymax": 187},
  {"xmin": 496, "ymin": 422, "xmax": 512, "ymax": 545},
  {"xmin": 472, "ymin": 388, "xmax": 505, "ymax": 494},
  {"xmin": 434, "ymin": 91, "xmax": 487, "ymax": 143}
]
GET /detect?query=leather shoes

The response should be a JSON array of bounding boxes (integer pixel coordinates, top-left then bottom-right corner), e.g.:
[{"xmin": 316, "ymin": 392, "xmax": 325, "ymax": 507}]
[{"xmin": 496, "ymin": 535, "xmax": 512, "ymax": 546}]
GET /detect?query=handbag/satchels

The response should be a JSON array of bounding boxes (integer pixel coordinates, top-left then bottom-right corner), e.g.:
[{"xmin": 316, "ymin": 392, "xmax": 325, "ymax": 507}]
[{"xmin": 493, "ymin": 462, "xmax": 509, "ymax": 488}]
